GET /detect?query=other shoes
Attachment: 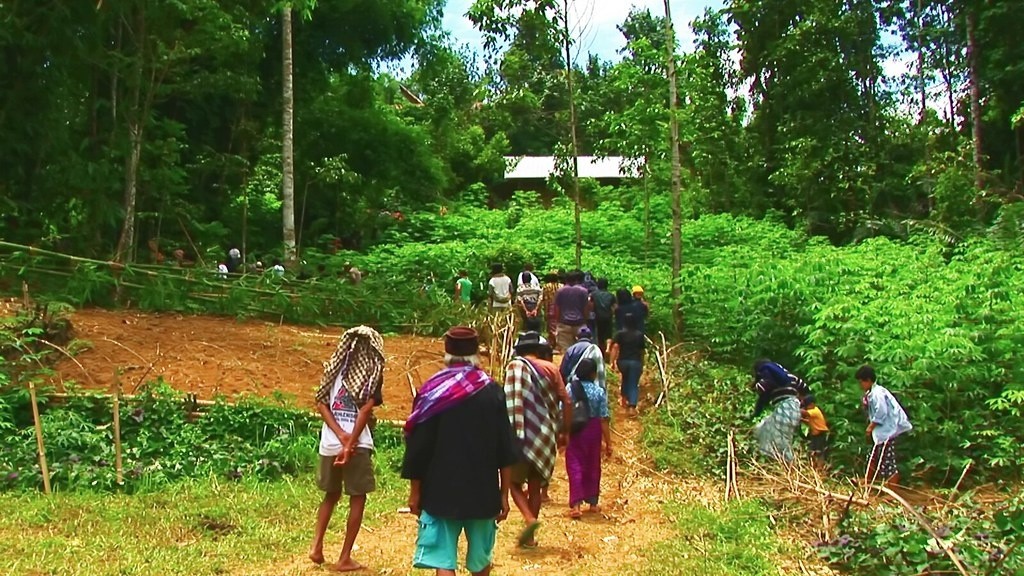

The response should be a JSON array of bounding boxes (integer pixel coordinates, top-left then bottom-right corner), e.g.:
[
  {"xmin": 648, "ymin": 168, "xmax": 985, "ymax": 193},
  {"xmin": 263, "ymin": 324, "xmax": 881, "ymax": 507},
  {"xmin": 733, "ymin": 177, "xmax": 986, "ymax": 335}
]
[
  {"xmin": 629, "ymin": 410, "xmax": 640, "ymax": 419},
  {"xmin": 589, "ymin": 506, "xmax": 600, "ymax": 512},
  {"xmin": 570, "ymin": 508, "xmax": 583, "ymax": 518}
]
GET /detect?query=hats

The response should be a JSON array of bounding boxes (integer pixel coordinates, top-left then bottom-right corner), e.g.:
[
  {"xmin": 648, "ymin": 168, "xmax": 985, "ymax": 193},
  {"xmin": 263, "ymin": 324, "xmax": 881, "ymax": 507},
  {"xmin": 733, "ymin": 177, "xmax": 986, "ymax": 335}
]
[
  {"xmin": 577, "ymin": 326, "xmax": 591, "ymax": 336},
  {"xmin": 632, "ymin": 285, "xmax": 644, "ymax": 296},
  {"xmin": 800, "ymin": 394, "xmax": 816, "ymax": 408},
  {"xmin": 513, "ymin": 329, "xmax": 553, "ymax": 355},
  {"xmin": 445, "ymin": 327, "xmax": 479, "ymax": 356},
  {"xmin": 490, "ymin": 262, "xmax": 505, "ymax": 273}
]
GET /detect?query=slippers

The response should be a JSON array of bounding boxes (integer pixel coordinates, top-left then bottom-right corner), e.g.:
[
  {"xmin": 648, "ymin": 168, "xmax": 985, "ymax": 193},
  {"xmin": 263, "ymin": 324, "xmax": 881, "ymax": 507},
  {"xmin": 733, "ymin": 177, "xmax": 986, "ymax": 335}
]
[
  {"xmin": 516, "ymin": 520, "xmax": 541, "ymax": 546},
  {"xmin": 522, "ymin": 540, "xmax": 538, "ymax": 549}
]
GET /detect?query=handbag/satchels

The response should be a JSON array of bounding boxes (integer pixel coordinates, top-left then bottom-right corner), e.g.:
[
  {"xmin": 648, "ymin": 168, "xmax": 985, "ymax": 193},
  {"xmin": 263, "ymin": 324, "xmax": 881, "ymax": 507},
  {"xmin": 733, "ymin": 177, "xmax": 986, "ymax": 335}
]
[{"xmin": 557, "ymin": 401, "xmax": 588, "ymax": 434}]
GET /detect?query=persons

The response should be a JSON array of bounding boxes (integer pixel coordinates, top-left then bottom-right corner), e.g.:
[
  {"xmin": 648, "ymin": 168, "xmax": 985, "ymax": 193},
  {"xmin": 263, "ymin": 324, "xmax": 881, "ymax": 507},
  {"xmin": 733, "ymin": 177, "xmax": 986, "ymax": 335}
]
[
  {"xmin": 399, "ymin": 326, "xmax": 517, "ymax": 576},
  {"xmin": 309, "ymin": 325, "xmax": 385, "ymax": 572},
  {"xmin": 439, "ymin": 205, "xmax": 447, "ymax": 216},
  {"xmin": 296, "ymin": 264, "xmax": 331, "ymax": 280},
  {"xmin": 344, "ymin": 262, "xmax": 361, "ymax": 283},
  {"xmin": 851, "ymin": 365, "xmax": 912, "ymax": 501},
  {"xmin": 252, "ymin": 253, "xmax": 285, "ymax": 277},
  {"xmin": 217, "ymin": 244, "xmax": 241, "ymax": 278},
  {"xmin": 502, "ymin": 330, "xmax": 573, "ymax": 548},
  {"xmin": 378, "ymin": 208, "xmax": 403, "ymax": 220},
  {"xmin": 455, "ymin": 263, "xmax": 649, "ymax": 456},
  {"xmin": 559, "ymin": 358, "xmax": 614, "ymax": 517},
  {"xmin": 750, "ymin": 356, "xmax": 830, "ymax": 467}
]
